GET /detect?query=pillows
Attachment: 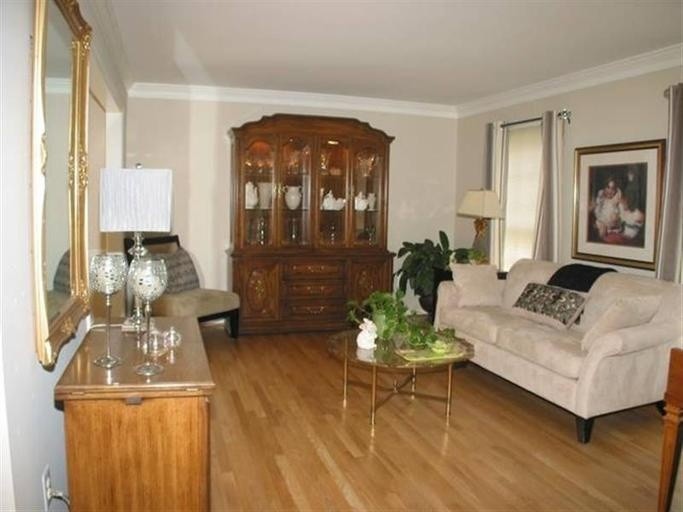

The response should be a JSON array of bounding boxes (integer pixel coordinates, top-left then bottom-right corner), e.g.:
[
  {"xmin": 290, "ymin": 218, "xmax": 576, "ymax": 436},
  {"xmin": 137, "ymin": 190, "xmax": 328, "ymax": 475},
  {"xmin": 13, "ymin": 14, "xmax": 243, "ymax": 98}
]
[
  {"xmin": 510, "ymin": 281, "xmax": 588, "ymax": 333},
  {"xmin": 53, "ymin": 249, "xmax": 70, "ymax": 296},
  {"xmin": 151, "ymin": 246, "xmax": 200, "ymax": 293},
  {"xmin": 449, "ymin": 263, "xmax": 502, "ymax": 309},
  {"xmin": 581, "ymin": 294, "xmax": 663, "ymax": 352}
]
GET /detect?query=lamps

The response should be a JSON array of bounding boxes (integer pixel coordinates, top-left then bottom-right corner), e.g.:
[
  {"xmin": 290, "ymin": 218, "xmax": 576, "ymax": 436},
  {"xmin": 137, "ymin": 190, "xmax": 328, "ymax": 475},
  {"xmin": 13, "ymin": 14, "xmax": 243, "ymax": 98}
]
[
  {"xmin": 98, "ymin": 163, "xmax": 174, "ymax": 324},
  {"xmin": 455, "ymin": 186, "xmax": 503, "ymax": 261}
]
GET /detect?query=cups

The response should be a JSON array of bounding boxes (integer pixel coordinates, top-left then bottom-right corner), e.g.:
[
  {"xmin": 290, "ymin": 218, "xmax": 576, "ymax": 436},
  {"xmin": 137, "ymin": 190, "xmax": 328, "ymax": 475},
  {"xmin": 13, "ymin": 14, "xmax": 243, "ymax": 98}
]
[
  {"xmin": 255, "ymin": 181, "xmax": 272, "ymax": 209},
  {"xmin": 283, "ymin": 185, "xmax": 301, "ymax": 210}
]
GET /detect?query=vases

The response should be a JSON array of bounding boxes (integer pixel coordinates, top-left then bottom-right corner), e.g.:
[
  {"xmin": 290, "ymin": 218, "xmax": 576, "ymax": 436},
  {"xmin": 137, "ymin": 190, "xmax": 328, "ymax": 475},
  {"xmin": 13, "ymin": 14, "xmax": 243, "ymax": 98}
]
[{"xmin": 243, "ymin": 179, "xmax": 303, "ymax": 212}]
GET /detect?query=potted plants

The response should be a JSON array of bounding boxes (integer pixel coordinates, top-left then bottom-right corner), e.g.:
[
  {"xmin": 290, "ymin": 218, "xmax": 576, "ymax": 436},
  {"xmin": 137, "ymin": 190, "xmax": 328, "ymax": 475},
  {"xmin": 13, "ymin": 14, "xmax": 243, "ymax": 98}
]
[
  {"xmin": 347, "ymin": 294, "xmax": 454, "ymax": 362},
  {"xmin": 394, "ymin": 230, "xmax": 469, "ymax": 323}
]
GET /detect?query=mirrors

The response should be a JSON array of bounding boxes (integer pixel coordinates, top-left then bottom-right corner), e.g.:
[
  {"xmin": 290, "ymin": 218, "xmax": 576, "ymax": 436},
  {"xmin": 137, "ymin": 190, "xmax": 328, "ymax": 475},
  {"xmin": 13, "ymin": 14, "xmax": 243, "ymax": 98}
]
[{"xmin": 31, "ymin": 0, "xmax": 92, "ymax": 370}]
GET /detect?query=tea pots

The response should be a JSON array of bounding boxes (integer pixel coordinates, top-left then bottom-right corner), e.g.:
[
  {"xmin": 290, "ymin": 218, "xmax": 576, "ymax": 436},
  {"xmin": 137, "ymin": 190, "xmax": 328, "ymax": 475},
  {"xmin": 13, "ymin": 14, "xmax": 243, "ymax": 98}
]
[
  {"xmin": 320, "ymin": 187, "xmax": 376, "ymax": 212},
  {"xmin": 244, "ymin": 181, "xmax": 258, "ymax": 210}
]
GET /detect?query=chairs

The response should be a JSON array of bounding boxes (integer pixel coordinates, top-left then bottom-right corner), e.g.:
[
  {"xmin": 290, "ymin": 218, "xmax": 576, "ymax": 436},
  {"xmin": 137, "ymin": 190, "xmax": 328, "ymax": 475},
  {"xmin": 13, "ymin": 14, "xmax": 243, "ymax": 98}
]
[{"xmin": 124, "ymin": 234, "xmax": 241, "ymax": 343}]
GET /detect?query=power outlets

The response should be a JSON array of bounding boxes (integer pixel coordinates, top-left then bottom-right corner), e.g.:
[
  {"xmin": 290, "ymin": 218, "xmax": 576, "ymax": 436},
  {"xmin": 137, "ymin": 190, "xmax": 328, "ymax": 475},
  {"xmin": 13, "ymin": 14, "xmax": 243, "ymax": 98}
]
[{"xmin": 40, "ymin": 463, "xmax": 54, "ymax": 511}]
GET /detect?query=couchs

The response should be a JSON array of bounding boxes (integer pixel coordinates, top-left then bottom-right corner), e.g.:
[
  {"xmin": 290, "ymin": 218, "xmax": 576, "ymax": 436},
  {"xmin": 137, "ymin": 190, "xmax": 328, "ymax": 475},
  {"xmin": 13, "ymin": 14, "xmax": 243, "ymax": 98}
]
[{"xmin": 433, "ymin": 258, "xmax": 683, "ymax": 445}]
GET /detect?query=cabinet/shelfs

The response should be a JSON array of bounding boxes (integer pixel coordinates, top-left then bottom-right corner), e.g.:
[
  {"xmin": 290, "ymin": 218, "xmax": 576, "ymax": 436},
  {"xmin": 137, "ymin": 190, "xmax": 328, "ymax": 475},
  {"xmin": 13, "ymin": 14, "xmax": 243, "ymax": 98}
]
[
  {"xmin": 55, "ymin": 317, "xmax": 219, "ymax": 512},
  {"xmin": 224, "ymin": 113, "xmax": 397, "ymax": 338}
]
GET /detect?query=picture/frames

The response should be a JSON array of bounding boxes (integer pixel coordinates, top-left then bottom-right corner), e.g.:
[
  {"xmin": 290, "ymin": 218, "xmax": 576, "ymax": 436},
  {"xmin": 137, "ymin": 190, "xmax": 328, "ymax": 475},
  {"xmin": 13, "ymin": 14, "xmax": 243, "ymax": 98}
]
[{"xmin": 571, "ymin": 138, "xmax": 667, "ymax": 273}]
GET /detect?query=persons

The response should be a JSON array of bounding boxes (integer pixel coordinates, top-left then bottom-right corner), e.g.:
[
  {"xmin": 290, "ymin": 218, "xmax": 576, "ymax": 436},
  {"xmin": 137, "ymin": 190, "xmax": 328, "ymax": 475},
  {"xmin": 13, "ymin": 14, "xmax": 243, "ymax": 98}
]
[{"xmin": 588, "ymin": 165, "xmax": 644, "ymax": 239}]
